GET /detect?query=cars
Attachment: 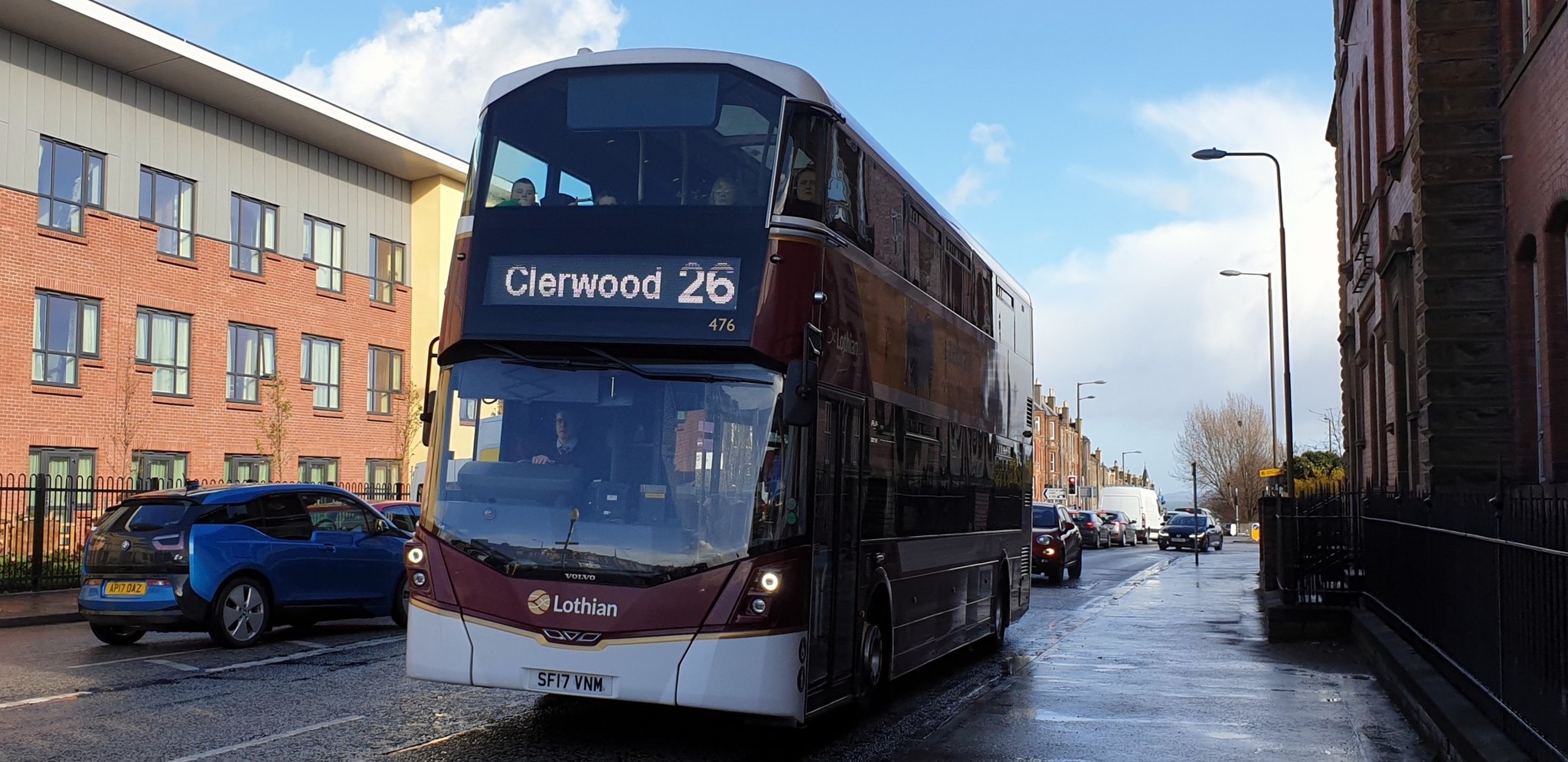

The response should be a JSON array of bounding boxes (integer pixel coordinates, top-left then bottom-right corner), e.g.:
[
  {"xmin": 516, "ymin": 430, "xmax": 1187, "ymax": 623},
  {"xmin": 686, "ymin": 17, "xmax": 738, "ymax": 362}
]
[
  {"xmin": 1158, "ymin": 507, "xmax": 1224, "ymax": 552},
  {"xmin": 78, "ymin": 484, "xmax": 422, "ymax": 648}
]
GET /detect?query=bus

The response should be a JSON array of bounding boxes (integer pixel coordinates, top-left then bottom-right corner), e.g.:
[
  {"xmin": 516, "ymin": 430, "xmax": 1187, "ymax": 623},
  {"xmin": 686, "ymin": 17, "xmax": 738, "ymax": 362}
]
[{"xmin": 405, "ymin": 47, "xmax": 1036, "ymax": 726}]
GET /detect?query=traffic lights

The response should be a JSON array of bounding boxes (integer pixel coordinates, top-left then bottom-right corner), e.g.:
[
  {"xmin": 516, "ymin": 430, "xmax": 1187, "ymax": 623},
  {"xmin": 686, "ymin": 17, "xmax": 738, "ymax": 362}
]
[{"xmin": 1067, "ymin": 476, "xmax": 1077, "ymax": 496}]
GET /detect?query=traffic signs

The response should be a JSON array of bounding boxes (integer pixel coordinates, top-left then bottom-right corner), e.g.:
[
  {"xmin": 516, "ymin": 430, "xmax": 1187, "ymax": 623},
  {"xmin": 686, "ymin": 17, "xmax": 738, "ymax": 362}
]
[{"xmin": 1042, "ymin": 488, "xmax": 1067, "ymax": 500}]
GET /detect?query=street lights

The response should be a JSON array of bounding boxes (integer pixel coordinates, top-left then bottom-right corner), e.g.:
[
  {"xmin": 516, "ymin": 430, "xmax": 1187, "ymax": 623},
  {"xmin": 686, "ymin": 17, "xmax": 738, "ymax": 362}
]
[
  {"xmin": 1122, "ymin": 450, "xmax": 1142, "ymax": 486},
  {"xmin": 1192, "ymin": 148, "xmax": 1298, "ymax": 606},
  {"xmin": 1220, "ymin": 270, "xmax": 1284, "ymax": 588},
  {"xmin": 1324, "ymin": 417, "xmax": 1332, "ymax": 454},
  {"xmin": 1077, "ymin": 379, "xmax": 1107, "ymax": 509}
]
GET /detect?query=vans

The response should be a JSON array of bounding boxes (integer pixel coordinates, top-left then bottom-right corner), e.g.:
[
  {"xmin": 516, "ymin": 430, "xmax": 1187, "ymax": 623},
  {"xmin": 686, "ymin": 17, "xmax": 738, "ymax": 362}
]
[{"xmin": 1098, "ymin": 487, "xmax": 1168, "ymax": 545}]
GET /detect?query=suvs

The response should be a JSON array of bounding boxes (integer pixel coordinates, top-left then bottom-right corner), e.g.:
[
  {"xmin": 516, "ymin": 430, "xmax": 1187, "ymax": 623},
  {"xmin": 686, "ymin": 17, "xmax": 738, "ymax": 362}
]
[{"xmin": 1031, "ymin": 501, "xmax": 1137, "ymax": 585}]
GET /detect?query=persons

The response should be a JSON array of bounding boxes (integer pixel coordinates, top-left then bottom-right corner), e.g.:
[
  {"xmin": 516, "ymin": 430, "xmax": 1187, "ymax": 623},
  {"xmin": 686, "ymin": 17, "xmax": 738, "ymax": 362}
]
[
  {"xmin": 591, "ymin": 188, "xmax": 620, "ymax": 207},
  {"xmin": 495, "ymin": 177, "xmax": 541, "ymax": 209},
  {"xmin": 710, "ymin": 173, "xmax": 741, "ymax": 207},
  {"xmin": 532, "ymin": 407, "xmax": 605, "ymax": 479},
  {"xmin": 768, "ymin": 439, "xmax": 801, "ymax": 537},
  {"xmin": 789, "ymin": 163, "xmax": 821, "ymax": 210}
]
